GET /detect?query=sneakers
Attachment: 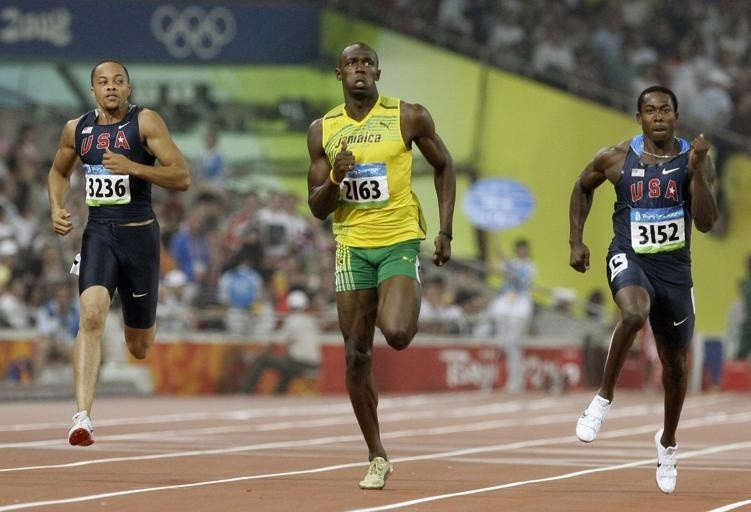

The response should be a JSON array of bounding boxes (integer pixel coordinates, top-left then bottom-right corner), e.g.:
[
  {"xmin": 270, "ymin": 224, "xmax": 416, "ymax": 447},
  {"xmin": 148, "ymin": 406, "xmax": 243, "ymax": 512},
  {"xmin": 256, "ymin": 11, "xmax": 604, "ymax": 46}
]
[
  {"xmin": 68, "ymin": 410, "xmax": 95, "ymax": 446},
  {"xmin": 575, "ymin": 395, "xmax": 612, "ymax": 443},
  {"xmin": 359, "ymin": 457, "xmax": 393, "ymax": 489},
  {"xmin": 655, "ymin": 429, "xmax": 678, "ymax": 494}
]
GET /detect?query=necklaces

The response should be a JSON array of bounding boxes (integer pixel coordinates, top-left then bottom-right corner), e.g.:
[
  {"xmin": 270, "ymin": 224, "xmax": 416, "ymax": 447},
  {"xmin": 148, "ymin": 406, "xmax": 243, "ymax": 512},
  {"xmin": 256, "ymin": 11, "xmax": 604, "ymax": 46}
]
[{"xmin": 644, "ymin": 150, "xmax": 678, "ymax": 158}]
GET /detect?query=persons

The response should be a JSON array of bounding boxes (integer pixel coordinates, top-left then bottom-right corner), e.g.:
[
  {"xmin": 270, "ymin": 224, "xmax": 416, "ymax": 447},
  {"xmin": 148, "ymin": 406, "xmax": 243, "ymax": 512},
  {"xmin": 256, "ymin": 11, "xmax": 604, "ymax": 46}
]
[
  {"xmin": 307, "ymin": 42, "xmax": 458, "ymax": 491},
  {"xmin": 567, "ymin": 82, "xmax": 718, "ymax": 494},
  {"xmin": 235, "ymin": 288, "xmax": 324, "ymax": 393},
  {"xmin": 48, "ymin": 61, "xmax": 193, "ymax": 448},
  {"xmin": 490, "ymin": 276, "xmax": 535, "ymax": 392},
  {"xmin": 576, "ymin": 252, "xmax": 751, "ymax": 398}
]
